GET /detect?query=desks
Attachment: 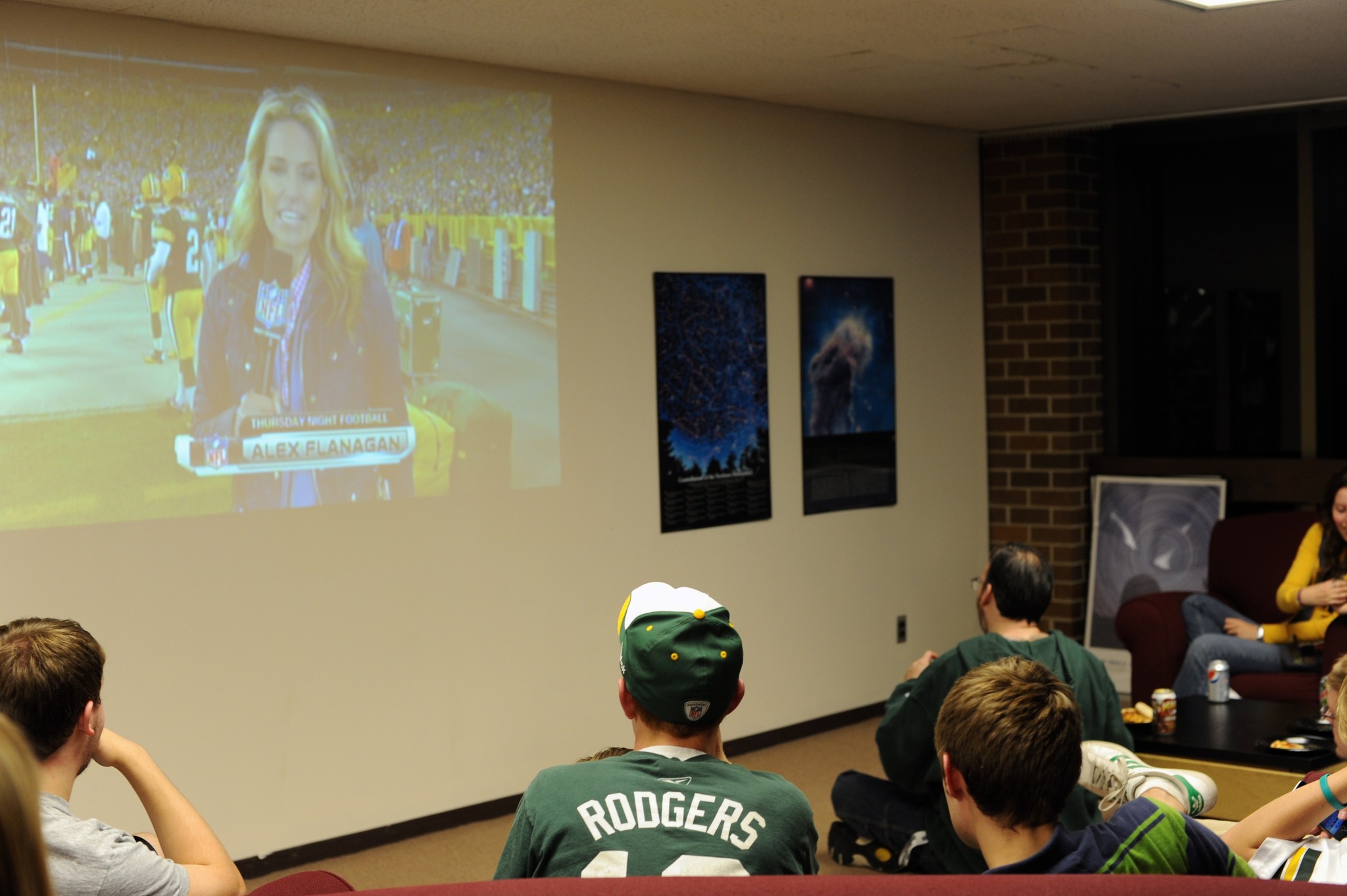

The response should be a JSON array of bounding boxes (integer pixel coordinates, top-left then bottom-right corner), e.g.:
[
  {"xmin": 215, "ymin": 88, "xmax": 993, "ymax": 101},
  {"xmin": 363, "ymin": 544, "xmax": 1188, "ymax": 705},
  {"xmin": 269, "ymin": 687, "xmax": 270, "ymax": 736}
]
[{"xmin": 1126, "ymin": 695, "xmax": 1347, "ymax": 822}]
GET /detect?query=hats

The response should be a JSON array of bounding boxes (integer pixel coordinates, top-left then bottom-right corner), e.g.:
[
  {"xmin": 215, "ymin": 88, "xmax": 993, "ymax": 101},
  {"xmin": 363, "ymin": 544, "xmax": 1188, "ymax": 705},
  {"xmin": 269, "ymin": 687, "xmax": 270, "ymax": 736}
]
[{"xmin": 617, "ymin": 581, "xmax": 743, "ymax": 723}]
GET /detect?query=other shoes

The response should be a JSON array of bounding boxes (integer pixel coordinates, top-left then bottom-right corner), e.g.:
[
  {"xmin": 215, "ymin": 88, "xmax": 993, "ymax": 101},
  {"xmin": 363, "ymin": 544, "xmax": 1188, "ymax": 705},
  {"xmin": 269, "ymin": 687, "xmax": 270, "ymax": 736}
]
[
  {"xmin": 1, "ymin": 274, "xmax": 65, "ymax": 354},
  {"xmin": 827, "ymin": 819, "xmax": 906, "ymax": 872}
]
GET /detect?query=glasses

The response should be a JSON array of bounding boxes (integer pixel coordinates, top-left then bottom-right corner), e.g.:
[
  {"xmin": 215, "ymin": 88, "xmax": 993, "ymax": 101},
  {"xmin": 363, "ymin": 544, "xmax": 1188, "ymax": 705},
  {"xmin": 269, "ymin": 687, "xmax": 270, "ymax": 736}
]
[
  {"xmin": 1320, "ymin": 705, "xmax": 1338, "ymax": 721},
  {"xmin": 971, "ymin": 576, "xmax": 992, "ymax": 589}
]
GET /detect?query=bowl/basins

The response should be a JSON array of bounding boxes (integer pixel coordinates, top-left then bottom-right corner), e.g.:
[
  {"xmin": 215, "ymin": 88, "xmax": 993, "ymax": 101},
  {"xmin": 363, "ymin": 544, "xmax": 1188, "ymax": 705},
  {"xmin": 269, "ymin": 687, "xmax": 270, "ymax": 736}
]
[{"xmin": 1123, "ymin": 717, "xmax": 1160, "ymax": 736}]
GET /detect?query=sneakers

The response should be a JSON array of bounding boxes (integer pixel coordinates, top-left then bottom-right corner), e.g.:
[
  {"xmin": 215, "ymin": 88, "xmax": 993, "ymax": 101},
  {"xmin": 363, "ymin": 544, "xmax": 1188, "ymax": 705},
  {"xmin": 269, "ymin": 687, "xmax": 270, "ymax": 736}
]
[
  {"xmin": 1077, "ymin": 741, "xmax": 1217, "ymax": 817},
  {"xmin": 144, "ymin": 350, "xmax": 165, "ymax": 363},
  {"xmin": 173, "ymin": 386, "xmax": 199, "ymax": 409}
]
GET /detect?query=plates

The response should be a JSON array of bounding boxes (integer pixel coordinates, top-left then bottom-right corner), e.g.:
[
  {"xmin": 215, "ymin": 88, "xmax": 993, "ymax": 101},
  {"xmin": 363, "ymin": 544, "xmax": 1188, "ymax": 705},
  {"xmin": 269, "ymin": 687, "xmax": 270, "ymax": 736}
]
[{"xmin": 1261, "ymin": 734, "xmax": 1332, "ymax": 758}]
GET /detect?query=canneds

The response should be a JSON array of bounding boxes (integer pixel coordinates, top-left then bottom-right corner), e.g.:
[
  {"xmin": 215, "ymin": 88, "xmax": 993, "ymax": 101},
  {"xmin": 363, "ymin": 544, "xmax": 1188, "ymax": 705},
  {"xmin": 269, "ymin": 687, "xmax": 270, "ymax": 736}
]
[
  {"xmin": 1151, "ymin": 689, "xmax": 1177, "ymax": 735},
  {"xmin": 1207, "ymin": 659, "xmax": 1229, "ymax": 703}
]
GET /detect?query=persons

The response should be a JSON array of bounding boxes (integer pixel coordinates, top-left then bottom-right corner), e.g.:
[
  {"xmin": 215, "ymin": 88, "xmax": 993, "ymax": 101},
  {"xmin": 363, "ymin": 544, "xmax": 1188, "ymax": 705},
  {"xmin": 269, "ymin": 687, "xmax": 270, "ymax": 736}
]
[
  {"xmin": 1218, "ymin": 652, "xmax": 1347, "ymax": 886},
  {"xmin": 494, "ymin": 582, "xmax": 821, "ymax": 878},
  {"xmin": 0, "ymin": 112, "xmax": 555, "ymax": 412},
  {"xmin": 195, "ymin": 94, "xmax": 414, "ymax": 509},
  {"xmin": 930, "ymin": 657, "xmax": 1261, "ymax": 879},
  {"xmin": 1172, "ymin": 471, "xmax": 1347, "ymax": 697},
  {"xmin": 1, "ymin": 616, "xmax": 357, "ymax": 895},
  {"xmin": 828, "ymin": 543, "xmax": 1135, "ymax": 874}
]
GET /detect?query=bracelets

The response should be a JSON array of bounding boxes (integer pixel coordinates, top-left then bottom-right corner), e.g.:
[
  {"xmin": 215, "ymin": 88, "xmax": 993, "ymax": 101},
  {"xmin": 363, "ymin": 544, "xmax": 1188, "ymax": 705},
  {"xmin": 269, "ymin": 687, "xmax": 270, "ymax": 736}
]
[
  {"xmin": 1257, "ymin": 625, "xmax": 1264, "ymax": 642},
  {"xmin": 1298, "ymin": 590, "xmax": 1304, "ymax": 606},
  {"xmin": 1320, "ymin": 773, "xmax": 1347, "ymax": 811}
]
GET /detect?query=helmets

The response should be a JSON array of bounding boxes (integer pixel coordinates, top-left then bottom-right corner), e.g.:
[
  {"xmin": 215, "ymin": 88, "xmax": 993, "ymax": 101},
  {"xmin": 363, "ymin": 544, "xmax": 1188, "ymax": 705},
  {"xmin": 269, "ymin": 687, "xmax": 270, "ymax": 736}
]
[
  {"xmin": 140, "ymin": 173, "xmax": 162, "ymax": 201},
  {"xmin": 161, "ymin": 164, "xmax": 188, "ymax": 205}
]
